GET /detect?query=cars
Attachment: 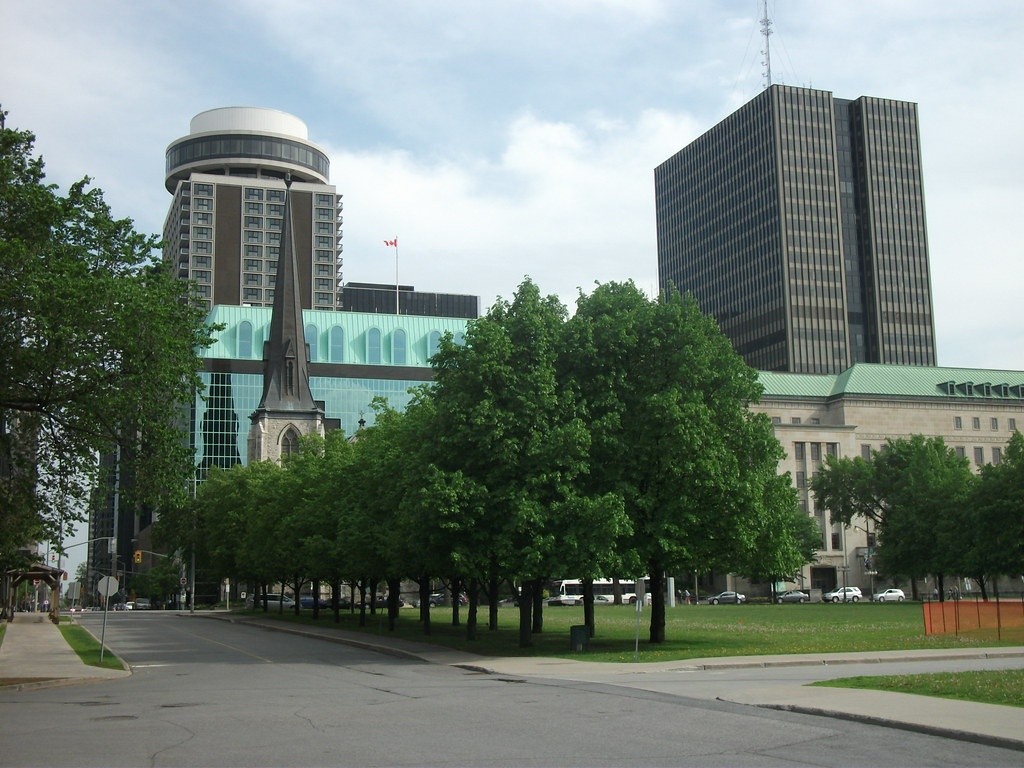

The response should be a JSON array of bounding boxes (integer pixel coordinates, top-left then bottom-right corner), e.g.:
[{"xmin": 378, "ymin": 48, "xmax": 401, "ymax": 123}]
[
  {"xmin": 869, "ymin": 588, "xmax": 905, "ymax": 603},
  {"xmin": 768, "ymin": 590, "xmax": 809, "ymax": 603},
  {"xmin": 296, "ymin": 595, "xmax": 405, "ymax": 610},
  {"xmin": 412, "ymin": 593, "xmax": 468, "ymax": 609},
  {"xmin": 117, "ymin": 597, "xmax": 152, "ymax": 610},
  {"xmin": 822, "ymin": 587, "xmax": 863, "ymax": 603},
  {"xmin": 708, "ymin": 591, "xmax": 746, "ymax": 604}
]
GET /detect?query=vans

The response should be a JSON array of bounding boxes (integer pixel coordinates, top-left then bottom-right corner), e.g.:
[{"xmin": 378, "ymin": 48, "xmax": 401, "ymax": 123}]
[{"xmin": 245, "ymin": 593, "xmax": 303, "ymax": 611}]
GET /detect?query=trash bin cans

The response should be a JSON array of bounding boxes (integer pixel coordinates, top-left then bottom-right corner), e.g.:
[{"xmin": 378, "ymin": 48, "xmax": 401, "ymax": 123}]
[{"xmin": 570, "ymin": 625, "xmax": 591, "ymax": 653}]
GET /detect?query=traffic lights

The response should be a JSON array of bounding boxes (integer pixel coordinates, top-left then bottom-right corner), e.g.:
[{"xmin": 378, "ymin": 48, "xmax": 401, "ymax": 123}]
[
  {"xmin": 135, "ymin": 550, "xmax": 142, "ymax": 564},
  {"xmin": 52, "ymin": 555, "xmax": 55, "ymax": 562}
]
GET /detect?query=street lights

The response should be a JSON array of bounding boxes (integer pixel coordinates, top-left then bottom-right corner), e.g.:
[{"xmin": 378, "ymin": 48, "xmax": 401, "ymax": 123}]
[{"xmin": 853, "ymin": 525, "xmax": 875, "ymax": 602}]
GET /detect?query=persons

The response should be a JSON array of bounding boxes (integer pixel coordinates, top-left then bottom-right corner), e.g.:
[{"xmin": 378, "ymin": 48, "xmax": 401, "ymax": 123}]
[
  {"xmin": 934, "ymin": 585, "xmax": 962, "ymax": 600},
  {"xmin": 677, "ymin": 589, "xmax": 691, "ymax": 604}
]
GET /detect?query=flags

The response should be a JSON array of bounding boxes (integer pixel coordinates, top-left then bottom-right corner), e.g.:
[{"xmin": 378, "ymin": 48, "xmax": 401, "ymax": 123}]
[{"xmin": 384, "ymin": 239, "xmax": 397, "ymax": 246}]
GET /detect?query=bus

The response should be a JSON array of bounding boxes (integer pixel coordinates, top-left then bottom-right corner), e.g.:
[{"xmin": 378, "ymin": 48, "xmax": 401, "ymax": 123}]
[{"xmin": 549, "ymin": 577, "xmax": 653, "ymax": 606}]
